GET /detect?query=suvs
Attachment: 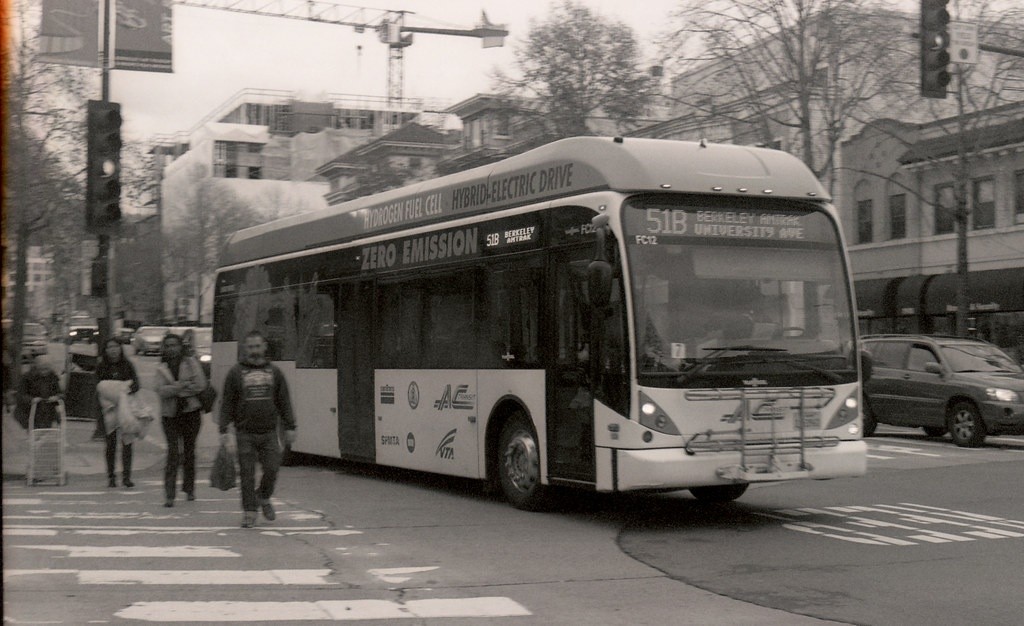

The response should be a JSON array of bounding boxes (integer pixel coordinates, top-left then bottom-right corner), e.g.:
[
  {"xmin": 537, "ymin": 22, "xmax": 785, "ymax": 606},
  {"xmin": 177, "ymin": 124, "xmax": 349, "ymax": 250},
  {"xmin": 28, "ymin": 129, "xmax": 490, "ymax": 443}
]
[{"xmin": 60, "ymin": 314, "xmax": 100, "ymax": 345}]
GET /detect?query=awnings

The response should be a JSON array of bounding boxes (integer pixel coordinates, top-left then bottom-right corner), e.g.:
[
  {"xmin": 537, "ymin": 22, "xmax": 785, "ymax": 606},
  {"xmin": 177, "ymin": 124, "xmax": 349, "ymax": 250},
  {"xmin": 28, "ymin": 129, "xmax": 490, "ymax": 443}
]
[{"xmin": 834, "ymin": 267, "xmax": 1024, "ymax": 321}]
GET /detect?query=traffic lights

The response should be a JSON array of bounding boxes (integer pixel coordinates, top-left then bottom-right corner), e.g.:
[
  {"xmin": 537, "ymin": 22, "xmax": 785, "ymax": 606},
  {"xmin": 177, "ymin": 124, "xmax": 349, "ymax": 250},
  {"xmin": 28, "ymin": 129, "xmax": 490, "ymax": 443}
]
[
  {"xmin": 83, "ymin": 98, "xmax": 126, "ymax": 237},
  {"xmin": 920, "ymin": 0, "xmax": 953, "ymax": 100}
]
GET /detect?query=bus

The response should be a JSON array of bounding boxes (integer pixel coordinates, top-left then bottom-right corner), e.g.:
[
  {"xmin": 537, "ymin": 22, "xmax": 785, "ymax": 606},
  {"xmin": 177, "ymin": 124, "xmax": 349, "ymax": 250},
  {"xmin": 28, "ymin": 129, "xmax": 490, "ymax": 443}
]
[{"xmin": 205, "ymin": 134, "xmax": 871, "ymax": 510}]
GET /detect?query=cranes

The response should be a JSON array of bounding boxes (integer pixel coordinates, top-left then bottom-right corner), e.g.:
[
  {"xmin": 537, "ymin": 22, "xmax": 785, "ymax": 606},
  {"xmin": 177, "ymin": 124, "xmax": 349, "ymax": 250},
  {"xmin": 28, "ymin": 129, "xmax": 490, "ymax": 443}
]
[{"xmin": 159, "ymin": 0, "xmax": 512, "ymax": 141}]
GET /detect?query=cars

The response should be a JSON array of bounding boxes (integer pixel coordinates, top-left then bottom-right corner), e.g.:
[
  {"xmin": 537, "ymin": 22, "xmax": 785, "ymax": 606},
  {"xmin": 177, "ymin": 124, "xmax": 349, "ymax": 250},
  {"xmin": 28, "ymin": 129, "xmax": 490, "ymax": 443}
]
[
  {"xmin": 855, "ymin": 333, "xmax": 1024, "ymax": 446},
  {"xmin": 180, "ymin": 327, "xmax": 213, "ymax": 375},
  {"xmin": 115, "ymin": 327, "xmax": 134, "ymax": 345},
  {"xmin": 7, "ymin": 323, "xmax": 48, "ymax": 356},
  {"xmin": 131, "ymin": 325, "xmax": 171, "ymax": 357},
  {"xmin": 1, "ymin": 319, "xmax": 15, "ymax": 334},
  {"xmin": 164, "ymin": 326, "xmax": 196, "ymax": 339}
]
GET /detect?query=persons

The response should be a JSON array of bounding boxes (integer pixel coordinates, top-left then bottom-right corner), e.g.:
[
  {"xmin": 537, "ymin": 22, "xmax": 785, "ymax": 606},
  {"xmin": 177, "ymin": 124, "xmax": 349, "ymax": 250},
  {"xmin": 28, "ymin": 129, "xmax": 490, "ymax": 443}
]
[
  {"xmin": 12, "ymin": 353, "xmax": 65, "ymax": 432},
  {"xmin": 218, "ymin": 331, "xmax": 297, "ymax": 528},
  {"xmin": 154, "ymin": 333, "xmax": 208, "ymax": 508},
  {"xmin": 93, "ymin": 337, "xmax": 141, "ymax": 488},
  {"xmin": 729, "ymin": 286, "xmax": 771, "ymax": 340}
]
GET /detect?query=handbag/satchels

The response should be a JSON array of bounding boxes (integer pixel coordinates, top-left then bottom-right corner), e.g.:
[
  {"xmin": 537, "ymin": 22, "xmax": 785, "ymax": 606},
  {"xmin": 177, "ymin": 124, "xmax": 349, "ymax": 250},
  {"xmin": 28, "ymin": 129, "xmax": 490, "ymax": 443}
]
[
  {"xmin": 196, "ymin": 385, "xmax": 216, "ymax": 413},
  {"xmin": 210, "ymin": 446, "xmax": 237, "ymax": 491}
]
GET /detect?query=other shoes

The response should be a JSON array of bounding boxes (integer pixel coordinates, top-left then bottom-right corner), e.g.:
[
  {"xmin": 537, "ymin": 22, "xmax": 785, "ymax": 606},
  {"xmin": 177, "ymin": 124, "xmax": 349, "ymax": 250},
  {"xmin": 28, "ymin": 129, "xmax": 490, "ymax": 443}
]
[
  {"xmin": 262, "ymin": 504, "xmax": 275, "ymax": 521},
  {"xmin": 109, "ymin": 479, "xmax": 115, "ymax": 487},
  {"xmin": 187, "ymin": 493, "xmax": 194, "ymax": 500},
  {"xmin": 165, "ymin": 498, "xmax": 173, "ymax": 507},
  {"xmin": 122, "ymin": 480, "xmax": 135, "ymax": 487},
  {"xmin": 240, "ymin": 511, "xmax": 257, "ymax": 527}
]
